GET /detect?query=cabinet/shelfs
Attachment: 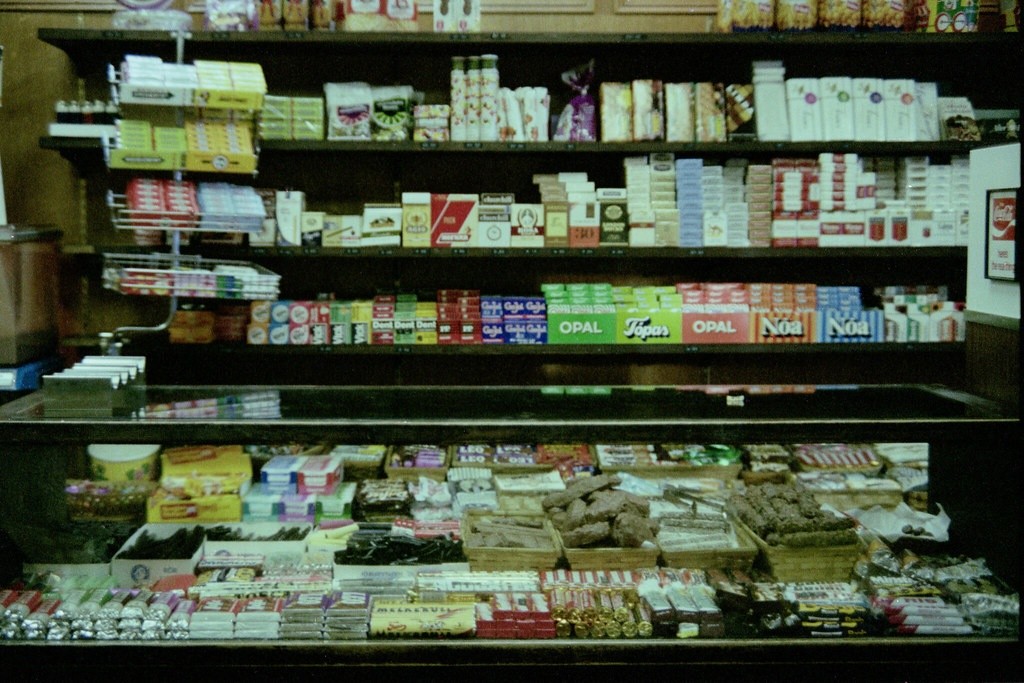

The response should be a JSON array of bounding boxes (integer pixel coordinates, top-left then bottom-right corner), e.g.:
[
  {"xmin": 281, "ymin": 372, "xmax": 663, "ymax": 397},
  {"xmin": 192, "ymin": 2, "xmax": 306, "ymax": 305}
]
[{"xmin": 35, "ymin": 26, "xmax": 1024, "ymax": 363}]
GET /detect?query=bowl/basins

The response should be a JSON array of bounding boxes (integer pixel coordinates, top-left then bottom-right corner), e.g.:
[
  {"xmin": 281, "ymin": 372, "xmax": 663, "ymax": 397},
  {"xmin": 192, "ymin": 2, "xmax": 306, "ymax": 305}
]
[{"xmin": 88, "ymin": 444, "xmax": 160, "ymax": 481}]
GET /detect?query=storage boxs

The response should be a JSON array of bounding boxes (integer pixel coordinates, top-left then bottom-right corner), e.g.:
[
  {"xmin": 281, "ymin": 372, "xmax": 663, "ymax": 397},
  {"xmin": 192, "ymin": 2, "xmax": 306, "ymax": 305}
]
[
  {"xmin": 532, "ymin": 592, "xmax": 551, "ymax": 620},
  {"xmin": 491, "ymin": 592, "xmax": 512, "ymax": 620},
  {"xmin": 277, "ymin": 494, "xmax": 316, "ymax": 515},
  {"xmin": 260, "ymin": 455, "xmax": 306, "ymax": 496},
  {"xmin": 307, "ymin": 525, "xmax": 348, "ymax": 554},
  {"xmin": 509, "ymin": 591, "xmax": 532, "ymax": 620},
  {"xmin": 515, "ymin": 621, "xmax": 556, "ymax": 640},
  {"xmin": 496, "ymin": 620, "xmax": 515, "ymax": 639},
  {"xmin": 475, "ymin": 603, "xmax": 495, "ymax": 637},
  {"xmin": 146, "ymin": 487, "xmax": 242, "ymax": 524},
  {"xmin": 332, "ymin": 539, "xmax": 470, "ymax": 579},
  {"xmin": 0, "ymin": 238, "xmax": 59, "ymax": 367},
  {"xmin": 22, "ymin": 537, "xmax": 112, "ymax": 577},
  {"xmin": 298, "ymin": 454, "xmax": 344, "ymax": 496},
  {"xmin": 109, "ymin": 0, "xmax": 976, "ymax": 345},
  {"xmin": 241, "ymin": 482, "xmax": 280, "ymax": 521},
  {"xmin": 642, "ymin": 590, "xmax": 726, "ymax": 639},
  {"xmin": 315, "ymin": 482, "xmax": 357, "ymax": 525},
  {"xmin": 110, "ymin": 522, "xmax": 207, "ymax": 588},
  {"xmin": 160, "ymin": 452, "xmax": 253, "ymax": 495},
  {"xmin": 202, "ymin": 521, "xmax": 313, "ymax": 557}
]
